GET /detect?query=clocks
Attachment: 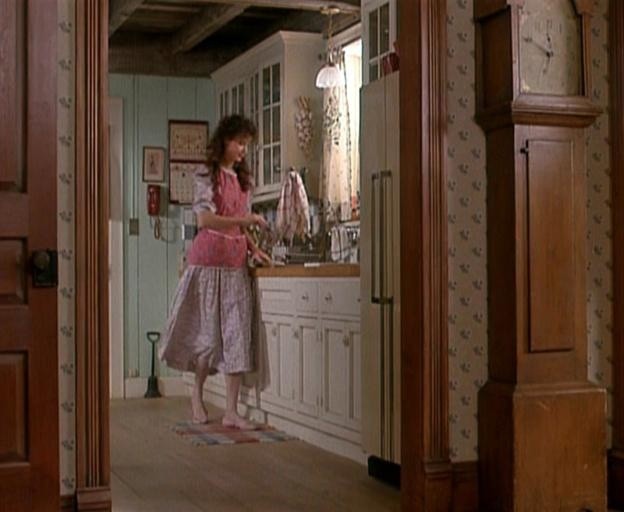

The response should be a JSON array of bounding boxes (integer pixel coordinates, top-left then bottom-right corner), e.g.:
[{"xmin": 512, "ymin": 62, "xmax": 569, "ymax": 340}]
[{"xmin": 472, "ymin": 0, "xmax": 611, "ymax": 512}]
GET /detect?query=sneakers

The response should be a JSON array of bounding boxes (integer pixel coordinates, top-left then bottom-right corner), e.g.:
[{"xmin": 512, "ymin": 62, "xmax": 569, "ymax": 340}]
[
  {"xmin": 192, "ymin": 411, "xmax": 210, "ymax": 426},
  {"xmin": 220, "ymin": 414, "xmax": 260, "ymax": 432}
]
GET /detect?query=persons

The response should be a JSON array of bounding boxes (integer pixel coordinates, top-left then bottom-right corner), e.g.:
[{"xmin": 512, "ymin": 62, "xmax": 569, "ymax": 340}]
[{"xmin": 154, "ymin": 112, "xmax": 275, "ymax": 432}]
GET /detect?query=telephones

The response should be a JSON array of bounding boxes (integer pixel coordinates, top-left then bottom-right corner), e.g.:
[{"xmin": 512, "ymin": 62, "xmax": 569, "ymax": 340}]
[{"xmin": 147, "ymin": 185, "xmax": 160, "ymax": 216}]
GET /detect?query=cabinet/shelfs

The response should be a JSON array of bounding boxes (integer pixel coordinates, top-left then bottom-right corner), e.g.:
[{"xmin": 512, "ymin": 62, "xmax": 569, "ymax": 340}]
[
  {"xmin": 210, "ymin": 30, "xmax": 325, "ymax": 202},
  {"xmin": 255, "ymin": 277, "xmax": 363, "ymax": 466},
  {"xmin": 360, "ymin": 1, "xmax": 398, "ymax": 83}
]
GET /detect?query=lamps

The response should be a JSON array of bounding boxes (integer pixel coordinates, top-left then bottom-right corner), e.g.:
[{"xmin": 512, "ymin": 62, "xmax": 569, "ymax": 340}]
[{"xmin": 313, "ymin": 6, "xmax": 343, "ymax": 88}]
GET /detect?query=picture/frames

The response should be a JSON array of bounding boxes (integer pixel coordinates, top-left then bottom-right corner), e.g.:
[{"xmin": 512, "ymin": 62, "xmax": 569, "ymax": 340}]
[
  {"xmin": 143, "ymin": 146, "xmax": 166, "ymax": 183},
  {"xmin": 168, "ymin": 120, "xmax": 210, "ymax": 204}
]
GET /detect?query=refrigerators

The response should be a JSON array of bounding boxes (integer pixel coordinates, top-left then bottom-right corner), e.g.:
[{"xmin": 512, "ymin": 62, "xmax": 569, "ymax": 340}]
[{"xmin": 359, "ymin": 73, "xmax": 401, "ymax": 465}]
